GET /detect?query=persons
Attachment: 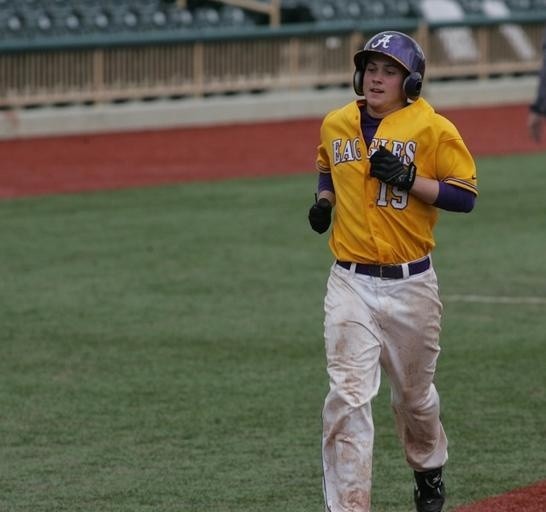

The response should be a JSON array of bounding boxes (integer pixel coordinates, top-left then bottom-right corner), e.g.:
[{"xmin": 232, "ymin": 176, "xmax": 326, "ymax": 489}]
[
  {"xmin": 309, "ymin": 31, "xmax": 479, "ymax": 512},
  {"xmin": 525, "ymin": 42, "xmax": 546, "ymax": 143}
]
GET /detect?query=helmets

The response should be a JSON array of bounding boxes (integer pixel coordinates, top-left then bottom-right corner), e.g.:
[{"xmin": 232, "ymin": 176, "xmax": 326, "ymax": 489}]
[{"xmin": 353, "ymin": 31, "xmax": 426, "ymax": 103}]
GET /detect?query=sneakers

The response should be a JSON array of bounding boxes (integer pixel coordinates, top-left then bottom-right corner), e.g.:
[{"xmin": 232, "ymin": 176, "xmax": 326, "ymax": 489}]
[{"xmin": 415, "ymin": 469, "xmax": 444, "ymax": 512}]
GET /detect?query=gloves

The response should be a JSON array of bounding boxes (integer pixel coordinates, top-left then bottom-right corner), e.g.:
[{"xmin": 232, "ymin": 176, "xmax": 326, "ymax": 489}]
[
  {"xmin": 309, "ymin": 197, "xmax": 332, "ymax": 235},
  {"xmin": 370, "ymin": 145, "xmax": 416, "ymax": 191}
]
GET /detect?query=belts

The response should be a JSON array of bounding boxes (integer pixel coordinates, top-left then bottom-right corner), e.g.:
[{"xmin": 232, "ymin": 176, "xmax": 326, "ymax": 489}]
[{"xmin": 336, "ymin": 257, "xmax": 430, "ymax": 279}]
